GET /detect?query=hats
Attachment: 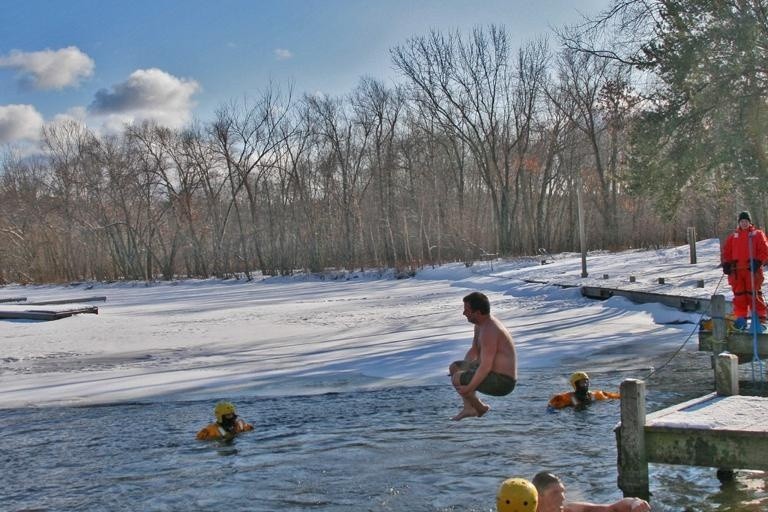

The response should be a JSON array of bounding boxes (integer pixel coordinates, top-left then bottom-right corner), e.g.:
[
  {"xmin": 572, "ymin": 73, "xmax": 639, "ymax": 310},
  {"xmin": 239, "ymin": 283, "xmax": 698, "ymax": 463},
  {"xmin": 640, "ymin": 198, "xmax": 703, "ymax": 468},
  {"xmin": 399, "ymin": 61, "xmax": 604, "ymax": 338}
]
[{"xmin": 738, "ymin": 212, "xmax": 750, "ymax": 222}]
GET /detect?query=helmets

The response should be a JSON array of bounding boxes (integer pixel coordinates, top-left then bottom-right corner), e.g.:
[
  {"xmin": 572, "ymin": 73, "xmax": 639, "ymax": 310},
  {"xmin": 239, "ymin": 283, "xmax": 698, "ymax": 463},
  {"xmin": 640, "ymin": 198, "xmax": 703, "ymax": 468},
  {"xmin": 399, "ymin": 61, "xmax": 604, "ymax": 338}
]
[
  {"xmin": 497, "ymin": 478, "xmax": 538, "ymax": 512},
  {"xmin": 215, "ymin": 402, "xmax": 235, "ymax": 424},
  {"xmin": 570, "ymin": 371, "xmax": 588, "ymax": 392}
]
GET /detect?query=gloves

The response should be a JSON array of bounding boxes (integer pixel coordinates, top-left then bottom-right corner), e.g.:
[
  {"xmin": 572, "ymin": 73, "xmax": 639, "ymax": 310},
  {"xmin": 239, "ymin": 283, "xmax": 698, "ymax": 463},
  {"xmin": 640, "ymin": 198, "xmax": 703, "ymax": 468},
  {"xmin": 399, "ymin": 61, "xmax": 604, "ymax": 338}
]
[
  {"xmin": 723, "ymin": 264, "xmax": 731, "ymax": 274},
  {"xmin": 749, "ymin": 260, "xmax": 760, "ymax": 273}
]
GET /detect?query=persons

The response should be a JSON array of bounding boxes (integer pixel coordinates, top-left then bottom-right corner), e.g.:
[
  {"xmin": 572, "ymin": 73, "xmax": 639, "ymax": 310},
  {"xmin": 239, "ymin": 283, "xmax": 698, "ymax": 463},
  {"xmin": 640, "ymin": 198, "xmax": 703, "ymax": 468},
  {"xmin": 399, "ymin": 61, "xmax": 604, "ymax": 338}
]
[
  {"xmin": 449, "ymin": 292, "xmax": 516, "ymax": 421},
  {"xmin": 195, "ymin": 402, "xmax": 253, "ymax": 440},
  {"xmin": 723, "ymin": 212, "xmax": 768, "ymax": 331},
  {"xmin": 549, "ymin": 372, "xmax": 620, "ymax": 408},
  {"xmin": 498, "ymin": 472, "xmax": 651, "ymax": 512}
]
